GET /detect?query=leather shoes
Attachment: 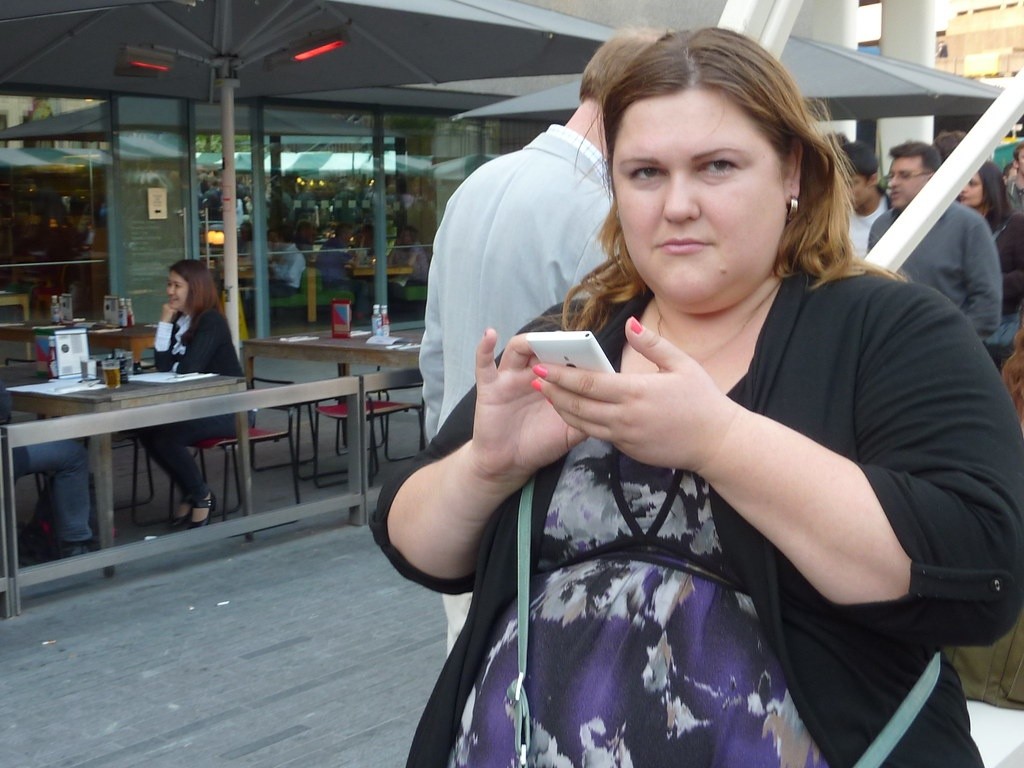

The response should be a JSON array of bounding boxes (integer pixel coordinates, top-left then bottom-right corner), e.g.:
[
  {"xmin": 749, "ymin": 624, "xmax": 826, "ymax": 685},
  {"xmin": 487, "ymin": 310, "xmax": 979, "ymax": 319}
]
[
  {"xmin": 169, "ymin": 498, "xmax": 194, "ymax": 531},
  {"xmin": 188, "ymin": 490, "xmax": 216, "ymax": 531}
]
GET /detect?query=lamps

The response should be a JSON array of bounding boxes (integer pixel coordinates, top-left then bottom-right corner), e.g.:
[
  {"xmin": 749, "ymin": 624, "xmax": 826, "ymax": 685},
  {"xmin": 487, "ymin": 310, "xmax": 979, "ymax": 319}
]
[
  {"xmin": 124, "ymin": 46, "xmax": 174, "ymax": 71},
  {"xmin": 288, "ymin": 30, "xmax": 349, "ymax": 62}
]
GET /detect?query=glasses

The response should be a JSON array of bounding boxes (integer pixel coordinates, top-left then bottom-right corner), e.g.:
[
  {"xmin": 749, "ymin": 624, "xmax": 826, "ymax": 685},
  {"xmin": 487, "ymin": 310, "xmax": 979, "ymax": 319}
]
[{"xmin": 886, "ymin": 170, "xmax": 931, "ymax": 179}]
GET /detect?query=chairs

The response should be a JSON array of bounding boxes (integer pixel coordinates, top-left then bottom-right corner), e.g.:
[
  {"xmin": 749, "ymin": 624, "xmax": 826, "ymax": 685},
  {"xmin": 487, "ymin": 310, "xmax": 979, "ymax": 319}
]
[
  {"xmin": 34, "ymin": 367, "xmax": 425, "ymax": 526},
  {"xmin": 168, "ymin": 378, "xmax": 300, "ymax": 525}
]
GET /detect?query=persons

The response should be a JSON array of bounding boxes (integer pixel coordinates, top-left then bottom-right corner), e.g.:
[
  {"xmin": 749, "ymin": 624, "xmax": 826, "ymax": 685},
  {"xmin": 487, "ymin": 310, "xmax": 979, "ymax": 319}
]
[
  {"xmin": 130, "ymin": 258, "xmax": 255, "ymax": 528},
  {"xmin": 367, "ymin": 24, "xmax": 1024, "ymax": 768},
  {"xmin": 415, "ymin": 23, "xmax": 664, "ymax": 659},
  {"xmin": 818, "ymin": 126, "xmax": 1024, "ymax": 438},
  {"xmin": 0, "ymin": 371, "xmax": 94, "ymax": 558},
  {"xmin": 230, "ymin": 219, "xmax": 430, "ymax": 327}
]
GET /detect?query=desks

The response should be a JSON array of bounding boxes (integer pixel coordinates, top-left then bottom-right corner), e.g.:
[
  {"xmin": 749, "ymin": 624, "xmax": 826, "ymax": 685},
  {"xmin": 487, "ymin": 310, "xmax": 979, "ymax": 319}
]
[
  {"xmin": 0, "ymin": 289, "xmax": 31, "ymax": 359},
  {"xmin": 242, "ymin": 330, "xmax": 423, "ymax": 470},
  {"xmin": 354, "ymin": 264, "xmax": 414, "ymax": 277},
  {"xmin": 3, "ymin": 377, "xmax": 252, "ymax": 551},
  {"xmin": 0, "ymin": 320, "xmax": 157, "ymax": 364}
]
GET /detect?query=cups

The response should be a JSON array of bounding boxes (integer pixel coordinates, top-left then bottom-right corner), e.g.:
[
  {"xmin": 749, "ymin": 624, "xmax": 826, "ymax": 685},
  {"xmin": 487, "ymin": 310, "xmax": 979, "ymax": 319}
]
[{"xmin": 102, "ymin": 360, "xmax": 121, "ymax": 389}]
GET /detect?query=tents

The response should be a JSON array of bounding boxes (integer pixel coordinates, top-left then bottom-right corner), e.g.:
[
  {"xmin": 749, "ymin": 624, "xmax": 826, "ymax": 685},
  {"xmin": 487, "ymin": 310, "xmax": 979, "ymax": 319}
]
[{"xmin": 193, "ymin": 149, "xmax": 505, "ymax": 185}]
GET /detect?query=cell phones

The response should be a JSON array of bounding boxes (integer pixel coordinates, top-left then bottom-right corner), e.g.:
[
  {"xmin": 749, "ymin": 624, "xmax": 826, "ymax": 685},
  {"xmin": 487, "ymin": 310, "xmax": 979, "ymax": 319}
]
[{"xmin": 527, "ymin": 330, "xmax": 615, "ymax": 373}]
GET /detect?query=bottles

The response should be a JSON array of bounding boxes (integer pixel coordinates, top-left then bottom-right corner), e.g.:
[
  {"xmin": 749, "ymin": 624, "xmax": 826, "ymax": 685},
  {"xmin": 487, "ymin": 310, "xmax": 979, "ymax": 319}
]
[
  {"xmin": 50, "ymin": 295, "xmax": 61, "ymax": 324},
  {"xmin": 371, "ymin": 304, "xmax": 390, "ymax": 336},
  {"xmin": 119, "ymin": 297, "xmax": 135, "ymax": 327}
]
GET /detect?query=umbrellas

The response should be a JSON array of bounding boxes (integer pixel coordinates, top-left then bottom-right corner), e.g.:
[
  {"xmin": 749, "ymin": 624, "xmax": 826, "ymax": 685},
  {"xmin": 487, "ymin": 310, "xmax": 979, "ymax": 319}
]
[
  {"xmin": 0, "ymin": 0, "xmax": 624, "ymax": 368},
  {"xmin": 449, "ymin": 31, "xmax": 1006, "ymax": 122}
]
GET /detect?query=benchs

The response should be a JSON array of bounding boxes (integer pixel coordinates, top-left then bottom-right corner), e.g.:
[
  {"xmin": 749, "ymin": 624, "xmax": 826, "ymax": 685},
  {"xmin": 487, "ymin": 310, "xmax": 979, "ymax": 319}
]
[{"xmin": 268, "ymin": 267, "xmax": 356, "ymax": 326}]
